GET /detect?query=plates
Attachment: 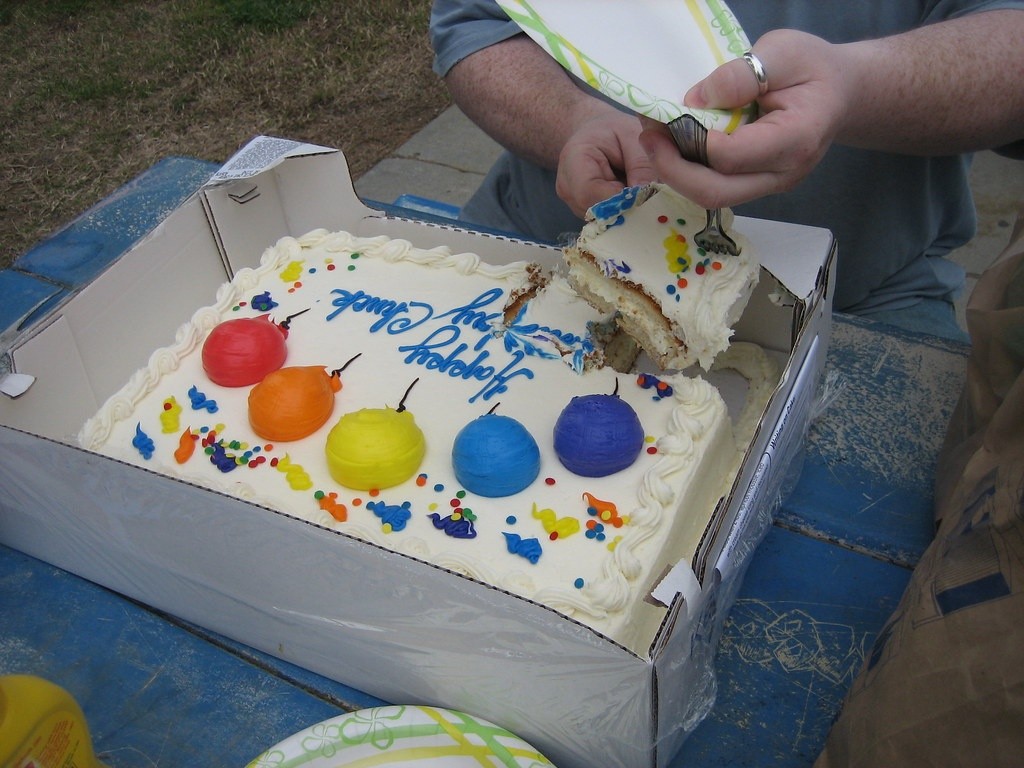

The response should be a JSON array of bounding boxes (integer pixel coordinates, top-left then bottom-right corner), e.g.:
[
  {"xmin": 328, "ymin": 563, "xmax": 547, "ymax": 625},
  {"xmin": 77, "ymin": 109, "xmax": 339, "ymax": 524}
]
[{"xmin": 243, "ymin": 702, "xmax": 558, "ymax": 768}]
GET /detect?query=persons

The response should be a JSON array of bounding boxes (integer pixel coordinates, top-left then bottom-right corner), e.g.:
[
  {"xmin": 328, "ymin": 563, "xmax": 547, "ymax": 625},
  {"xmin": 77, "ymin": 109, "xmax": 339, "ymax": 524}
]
[{"xmin": 428, "ymin": 0, "xmax": 1024, "ymax": 343}]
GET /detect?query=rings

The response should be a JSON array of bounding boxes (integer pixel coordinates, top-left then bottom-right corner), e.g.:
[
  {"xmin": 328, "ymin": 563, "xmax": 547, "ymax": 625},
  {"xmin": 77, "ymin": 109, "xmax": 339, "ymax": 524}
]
[{"xmin": 742, "ymin": 52, "xmax": 769, "ymax": 97}]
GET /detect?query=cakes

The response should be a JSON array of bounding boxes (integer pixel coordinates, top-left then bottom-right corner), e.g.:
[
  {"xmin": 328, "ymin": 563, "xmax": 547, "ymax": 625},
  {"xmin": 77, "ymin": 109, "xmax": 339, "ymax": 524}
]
[
  {"xmin": 78, "ymin": 228, "xmax": 786, "ymax": 660},
  {"xmin": 560, "ymin": 182, "xmax": 765, "ymax": 373}
]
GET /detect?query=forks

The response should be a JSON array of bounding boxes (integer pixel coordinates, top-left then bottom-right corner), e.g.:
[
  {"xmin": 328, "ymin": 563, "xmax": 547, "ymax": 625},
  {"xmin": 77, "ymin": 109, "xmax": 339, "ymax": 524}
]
[{"xmin": 666, "ymin": 114, "xmax": 737, "ymax": 257}]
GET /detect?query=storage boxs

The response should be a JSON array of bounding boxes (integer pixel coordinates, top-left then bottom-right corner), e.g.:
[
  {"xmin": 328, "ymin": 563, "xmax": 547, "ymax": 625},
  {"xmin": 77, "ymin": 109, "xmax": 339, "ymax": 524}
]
[{"xmin": 0, "ymin": 136, "xmax": 840, "ymax": 768}]
